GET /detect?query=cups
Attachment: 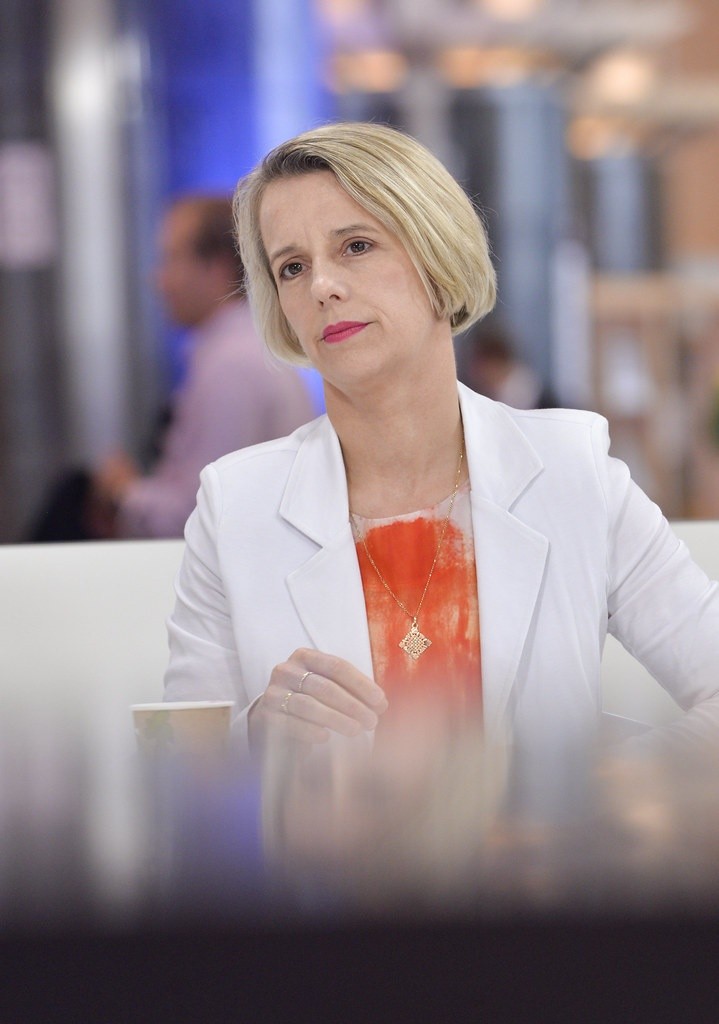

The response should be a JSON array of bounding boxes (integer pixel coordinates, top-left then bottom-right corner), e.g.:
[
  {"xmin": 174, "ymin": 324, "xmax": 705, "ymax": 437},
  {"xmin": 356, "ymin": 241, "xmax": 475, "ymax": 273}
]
[{"xmin": 131, "ymin": 699, "xmax": 238, "ymax": 804}]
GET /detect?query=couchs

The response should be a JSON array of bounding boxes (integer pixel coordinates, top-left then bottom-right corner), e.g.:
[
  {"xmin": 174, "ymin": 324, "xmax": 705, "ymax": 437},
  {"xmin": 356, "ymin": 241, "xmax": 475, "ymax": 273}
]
[{"xmin": 0, "ymin": 521, "xmax": 719, "ymax": 857}]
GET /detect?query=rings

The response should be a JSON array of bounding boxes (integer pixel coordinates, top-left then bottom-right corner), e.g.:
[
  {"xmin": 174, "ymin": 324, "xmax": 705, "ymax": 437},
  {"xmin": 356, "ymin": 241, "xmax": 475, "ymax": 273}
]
[
  {"xmin": 298, "ymin": 672, "xmax": 312, "ymax": 692},
  {"xmin": 280, "ymin": 693, "xmax": 292, "ymax": 714}
]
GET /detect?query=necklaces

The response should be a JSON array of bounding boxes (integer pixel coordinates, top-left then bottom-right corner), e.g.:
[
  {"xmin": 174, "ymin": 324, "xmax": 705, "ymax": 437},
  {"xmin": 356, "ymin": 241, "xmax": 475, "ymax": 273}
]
[{"xmin": 349, "ymin": 439, "xmax": 464, "ymax": 664}]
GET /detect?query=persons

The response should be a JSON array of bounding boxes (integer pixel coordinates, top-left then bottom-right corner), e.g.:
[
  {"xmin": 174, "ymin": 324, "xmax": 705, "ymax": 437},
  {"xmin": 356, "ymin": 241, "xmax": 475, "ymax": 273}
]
[
  {"xmin": 83, "ymin": 199, "xmax": 314, "ymax": 543},
  {"xmin": 162, "ymin": 121, "xmax": 718, "ymax": 913}
]
